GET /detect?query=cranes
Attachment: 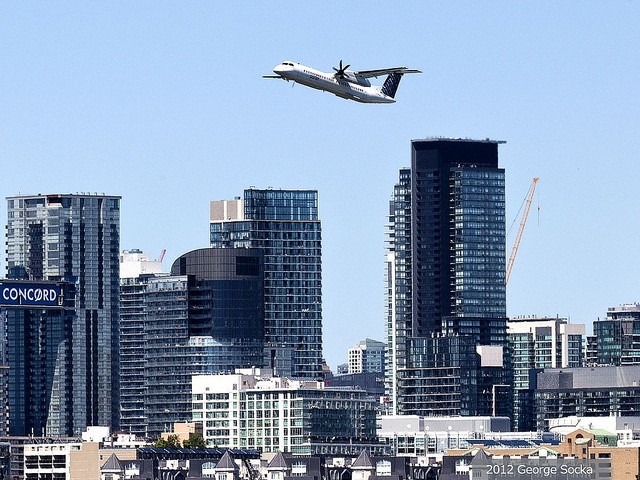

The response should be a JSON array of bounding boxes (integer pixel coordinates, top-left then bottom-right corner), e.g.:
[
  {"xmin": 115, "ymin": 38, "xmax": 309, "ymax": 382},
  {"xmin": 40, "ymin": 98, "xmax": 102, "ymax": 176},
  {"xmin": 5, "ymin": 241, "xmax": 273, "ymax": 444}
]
[{"xmin": 505, "ymin": 177, "xmax": 541, "ymax": 285}]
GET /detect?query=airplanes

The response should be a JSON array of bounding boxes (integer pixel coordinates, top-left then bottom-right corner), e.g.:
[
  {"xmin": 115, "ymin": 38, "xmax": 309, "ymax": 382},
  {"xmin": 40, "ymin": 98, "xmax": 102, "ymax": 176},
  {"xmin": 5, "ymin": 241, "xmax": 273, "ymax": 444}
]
[{"xmin": 262, "ymin": 60, "xmax": 423, "ymax": 103}]
